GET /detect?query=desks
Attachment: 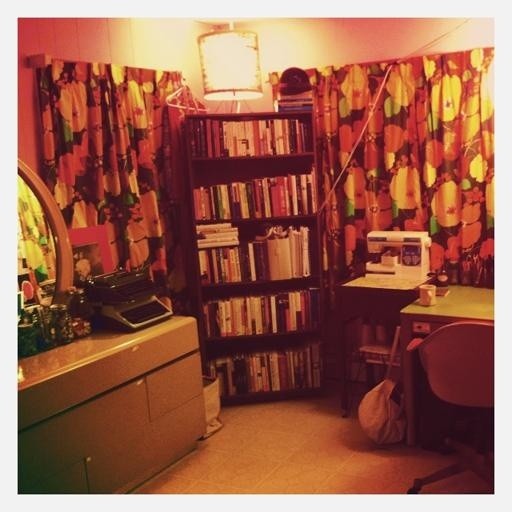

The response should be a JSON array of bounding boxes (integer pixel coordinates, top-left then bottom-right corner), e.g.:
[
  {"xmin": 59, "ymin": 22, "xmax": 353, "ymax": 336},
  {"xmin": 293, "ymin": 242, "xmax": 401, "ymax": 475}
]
[
  {"xmin": 333, "ymin": 273, "xmax": 438, "ymax": 416},
  {"xmin": 393, "ymin": 284, "xmax": 494, "ymax": 445},
  {"xmin": 17, "ymin": 317, "xmax": 206, "ymax": 494}
]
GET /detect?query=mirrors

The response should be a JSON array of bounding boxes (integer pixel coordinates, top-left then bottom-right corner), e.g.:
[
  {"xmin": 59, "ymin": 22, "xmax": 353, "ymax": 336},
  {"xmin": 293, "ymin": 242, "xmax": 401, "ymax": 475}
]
[{"xmin": 17, "ymin": 159, "xmax": 73, "ymax": 336}]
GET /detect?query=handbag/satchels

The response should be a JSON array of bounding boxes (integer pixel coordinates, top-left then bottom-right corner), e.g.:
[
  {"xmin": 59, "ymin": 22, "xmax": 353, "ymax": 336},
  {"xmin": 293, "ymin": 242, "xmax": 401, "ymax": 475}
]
[{"xmin": 357, "ymin": 378, "xmax": 408, "ymax": 450}]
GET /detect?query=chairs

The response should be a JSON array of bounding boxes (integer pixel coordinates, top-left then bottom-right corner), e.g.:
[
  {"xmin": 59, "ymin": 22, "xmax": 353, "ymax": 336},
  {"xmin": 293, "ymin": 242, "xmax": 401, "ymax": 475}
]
[{"xmin": 406, "ymin": 320, "xmax": 494, "ymax": 494}]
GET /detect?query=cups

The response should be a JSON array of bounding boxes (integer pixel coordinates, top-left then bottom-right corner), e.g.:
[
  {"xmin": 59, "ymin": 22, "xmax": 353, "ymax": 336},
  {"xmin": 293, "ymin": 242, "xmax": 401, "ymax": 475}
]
[
  {"xmin": 382, "ymin": 256, "xmax": 398, "ymax": 267},
  {"xmin": 420, "ymin": 284, "xmax": 436, "ymax": 307}
]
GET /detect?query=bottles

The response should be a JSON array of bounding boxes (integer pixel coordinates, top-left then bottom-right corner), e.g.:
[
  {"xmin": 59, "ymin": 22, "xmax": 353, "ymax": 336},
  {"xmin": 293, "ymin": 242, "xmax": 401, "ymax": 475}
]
[
  {"xmin": 19, "ymin": 293, "xmax": 91, "ymax": 360},
  {"xmin": 437, "ymin": 270, "xmax": 449, "ymax": 287}
]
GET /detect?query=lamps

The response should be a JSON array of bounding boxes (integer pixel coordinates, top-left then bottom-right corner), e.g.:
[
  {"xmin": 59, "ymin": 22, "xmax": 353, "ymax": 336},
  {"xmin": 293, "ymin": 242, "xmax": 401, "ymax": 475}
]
[{"xmin": 197, "ymin": 17, "xmax": 264, "ymax": 101}]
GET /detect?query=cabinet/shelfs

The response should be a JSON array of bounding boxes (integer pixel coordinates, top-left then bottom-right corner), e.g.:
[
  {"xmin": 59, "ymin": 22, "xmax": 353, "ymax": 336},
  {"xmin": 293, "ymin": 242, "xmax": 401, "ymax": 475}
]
[{"xmin": 183, "ymin": 109, "xmax": 327, "ymax": 405}]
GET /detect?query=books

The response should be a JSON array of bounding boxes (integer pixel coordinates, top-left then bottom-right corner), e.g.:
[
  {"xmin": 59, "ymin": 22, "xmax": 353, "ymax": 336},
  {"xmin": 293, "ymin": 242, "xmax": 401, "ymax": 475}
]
[{"xmin": 191, "ymin": 113, "xmax": 328, "ymax": 400}]
